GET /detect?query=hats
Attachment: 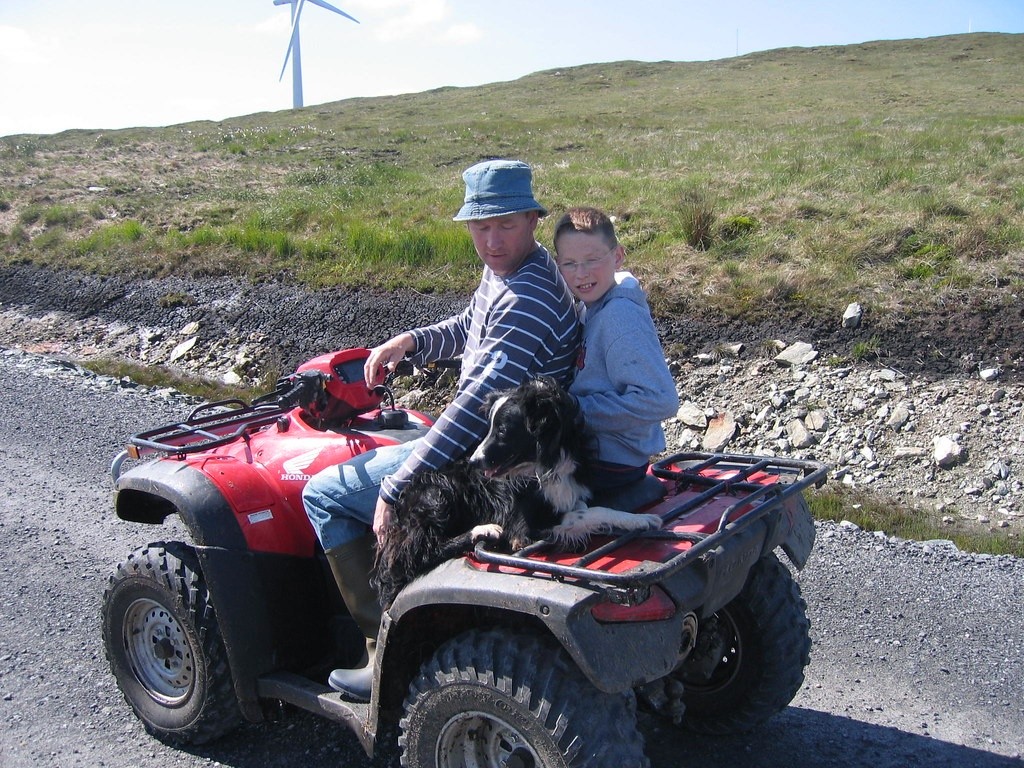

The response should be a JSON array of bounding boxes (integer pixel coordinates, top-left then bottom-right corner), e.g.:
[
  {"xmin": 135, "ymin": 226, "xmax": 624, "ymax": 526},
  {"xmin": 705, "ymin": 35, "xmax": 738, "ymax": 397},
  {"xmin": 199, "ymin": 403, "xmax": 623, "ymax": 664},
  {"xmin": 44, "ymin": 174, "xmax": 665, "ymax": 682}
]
[{"xmin": 452, "ymin": 160, "xmax": 547, "ymax": 222}]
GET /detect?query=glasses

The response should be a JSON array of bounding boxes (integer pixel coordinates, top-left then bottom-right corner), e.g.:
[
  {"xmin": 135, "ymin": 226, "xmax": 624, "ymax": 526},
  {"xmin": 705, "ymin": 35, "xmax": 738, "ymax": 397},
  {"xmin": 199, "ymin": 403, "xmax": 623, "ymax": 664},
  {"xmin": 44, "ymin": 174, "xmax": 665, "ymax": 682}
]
[{"xmin": 556, "ymin": 246, "xmax": 616, "ymax": 273}]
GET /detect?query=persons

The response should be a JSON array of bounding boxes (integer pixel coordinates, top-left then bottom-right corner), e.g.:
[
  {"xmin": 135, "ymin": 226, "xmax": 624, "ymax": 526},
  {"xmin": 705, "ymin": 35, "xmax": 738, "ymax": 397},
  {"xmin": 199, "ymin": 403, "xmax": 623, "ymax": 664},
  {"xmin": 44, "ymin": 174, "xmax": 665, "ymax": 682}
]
[
  {"xmin": 302, "ymin": 159, "xmax": 579, "ymax": 701},
  {"xmin": 539, "ymin": 206, "xmax": 679, "ymax": 507}
]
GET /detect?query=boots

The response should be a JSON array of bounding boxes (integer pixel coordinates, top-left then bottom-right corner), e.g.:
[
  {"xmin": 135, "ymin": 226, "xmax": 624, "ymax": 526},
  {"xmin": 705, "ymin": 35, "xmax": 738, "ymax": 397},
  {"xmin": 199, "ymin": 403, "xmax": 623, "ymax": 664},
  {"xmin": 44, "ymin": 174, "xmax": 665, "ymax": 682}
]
[{"xmin": 325, "ymin": 535, "xmax": 383, "ymax": 702}]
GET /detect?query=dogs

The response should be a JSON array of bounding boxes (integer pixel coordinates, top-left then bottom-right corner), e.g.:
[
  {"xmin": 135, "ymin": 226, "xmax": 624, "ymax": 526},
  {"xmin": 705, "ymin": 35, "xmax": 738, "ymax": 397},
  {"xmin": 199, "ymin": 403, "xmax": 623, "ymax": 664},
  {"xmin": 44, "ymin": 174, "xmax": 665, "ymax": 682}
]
[{"xmin": 369, "ymin": 374, "xmax": 662, "ymax": 613}]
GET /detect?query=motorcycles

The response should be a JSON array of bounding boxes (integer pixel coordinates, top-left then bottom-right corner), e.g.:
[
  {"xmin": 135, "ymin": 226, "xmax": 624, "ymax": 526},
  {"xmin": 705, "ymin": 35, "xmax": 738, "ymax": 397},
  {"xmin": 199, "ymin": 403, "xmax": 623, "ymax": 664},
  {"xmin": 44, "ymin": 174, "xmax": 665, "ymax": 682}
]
[{"xmin": 104, "ymin": 347, "xmax": 829, "ymax": 768}]
